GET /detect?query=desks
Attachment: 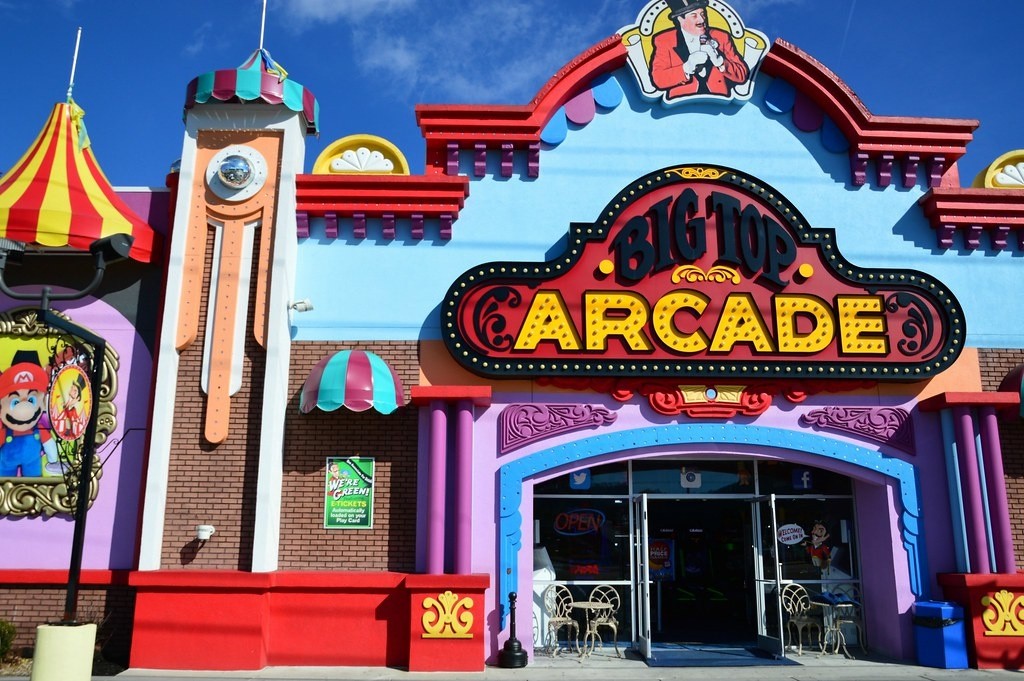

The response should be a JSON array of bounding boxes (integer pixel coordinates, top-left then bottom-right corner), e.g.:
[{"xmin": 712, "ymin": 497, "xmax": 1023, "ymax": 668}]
[
  {"xmin": 809, "ymin": 599, "xmax": 855, "ymax": 660},
  {"xmin": 567, "ymin": 602, "xmax": 614, "ymax": 663}
]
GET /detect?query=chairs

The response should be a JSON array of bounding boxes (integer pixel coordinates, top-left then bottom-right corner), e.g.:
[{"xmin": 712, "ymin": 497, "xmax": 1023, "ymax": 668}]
[
  {"xmin": 831, "ymin": 583, "xmax": 870, "ymax": 656},
  {"xmin": 782, "ymin": 584, "xmax": 826, "ymax": 657},
  {"xmin": 588, "ymin": 585, "xmax": 621, "ymax": 660},
  {"xmin": 542, "ymin": 583, "xmax": 581, "ymax": 658}
]
